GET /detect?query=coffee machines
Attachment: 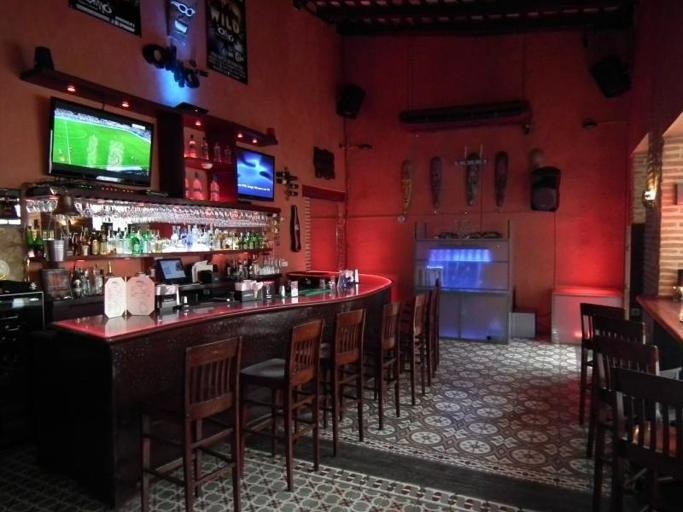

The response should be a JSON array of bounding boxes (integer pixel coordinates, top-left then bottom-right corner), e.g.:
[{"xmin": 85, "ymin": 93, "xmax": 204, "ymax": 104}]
[{"xmin": 191, "ymin": 260, "xmax": 214, "ymax": 284}]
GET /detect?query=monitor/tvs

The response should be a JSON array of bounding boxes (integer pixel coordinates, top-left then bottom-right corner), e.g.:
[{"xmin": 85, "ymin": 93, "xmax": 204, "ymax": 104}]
[
  {"xmin": 45, "ymin": 95, "xmax": 154, "ymax": 187},
  {"xmin": 156, "ymin": 258, "xmax": 188, "ymax": 282},
  {"xmin": 235, "ymin": 145, "xmax": 275, "ymax": 202}
]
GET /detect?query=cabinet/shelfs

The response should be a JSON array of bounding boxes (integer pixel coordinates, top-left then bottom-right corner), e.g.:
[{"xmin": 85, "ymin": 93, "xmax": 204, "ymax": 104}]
[
  {"xmin": 159, "ymin": 109, "xmax": 240, "ymax": 203},
  {"xmin": 405, "ymin": 218, "xmax": 515, "ymax": 346},
  {"xmin": 24, "ymin": 185, "xmax": 285, "ymax": 303}
]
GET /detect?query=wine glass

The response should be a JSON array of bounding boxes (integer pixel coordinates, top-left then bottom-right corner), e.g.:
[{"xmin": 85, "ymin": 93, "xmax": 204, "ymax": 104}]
[
  {"xmin": 26, "ymin": 199, "xmax": 56, "ymax": 214},
  {"xmin": 169, "ymin": 204, "xmax": 271, "ymax": 228},
  {"xmin": 73, "ymin": 198, "xmax": 169, "ymax": 224}
]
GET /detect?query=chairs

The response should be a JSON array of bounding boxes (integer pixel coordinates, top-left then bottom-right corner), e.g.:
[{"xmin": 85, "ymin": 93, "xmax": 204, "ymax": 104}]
[{"xmin": 574, "ymin": 303, "xmax": 683, "ymax": 512}]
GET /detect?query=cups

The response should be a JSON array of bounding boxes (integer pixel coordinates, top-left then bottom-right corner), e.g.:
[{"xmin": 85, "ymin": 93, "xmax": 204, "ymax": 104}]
[{"xmin": 46, "ymin": 239, "xmax": 65, "ymax": 262}]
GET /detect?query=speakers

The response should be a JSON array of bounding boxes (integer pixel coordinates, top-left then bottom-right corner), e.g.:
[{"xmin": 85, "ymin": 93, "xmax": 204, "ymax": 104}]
[
  {"xmin": 595, "ymin": 55, "xmax": 628, "ymax": 98},
  {"xmin": 336, "ymin": 83, "xmax": 365, "ymax": 119}
]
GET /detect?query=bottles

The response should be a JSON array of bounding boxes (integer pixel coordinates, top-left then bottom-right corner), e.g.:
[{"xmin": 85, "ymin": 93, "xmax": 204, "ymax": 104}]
[
  {"xmin": 184, "ymin": 173, "xmax": 189, "ymax": 197},
  {"xmin": 115, "ymin": 225, "xmax": 170, "ymax": 253},
  {"xmin": 201, "ymin": 137, "xmax": 209, "ymax": 160},
  {"xmin": 32, "ymin": 230, "xmax": 45, "ymax": 258},
  {"xmin": 354, "ymin": 269, "xmax": 360, "ymax": 284},
  {"xmin": 237, "ymin": 231, "xmax": 269, "ymax": 250},
  {"xmin": 226, "ymin": 255, "xmax": 282, "ymax": 281},
  {"xmin": 71, "ymin": 261, "xmax": 113, "ymax": 298},
  {"xmin": 214, "ymin": 140, "xmax": 221, "ymax": 162},
  {"xmin": 210, "ymin": 175, "xmax": 220, "ymax": 202},
  {"xmin": 290, "ymin": 204, "xmax": 302, "ymax": 252},
  {"xmin": 279, "ymin": 285, "xmax": 286, "ymax": 297},
  {"xmin": 188, "ymin": 134, "xmax": 197, "ymax": 157},
  {"xmin": 224, "ymin": 144, "xmax": 231, "ymax": 163},
  {"xmin": 171, "ymin": 225, "xmax": 237, "ymax": 252},
  {"xmin": 192, "ymin": 171, "xmax": 203, "ymax": 200},
  {"xmin": 71, "ymin": 224, "xmax": 114, "ymax": 256}
]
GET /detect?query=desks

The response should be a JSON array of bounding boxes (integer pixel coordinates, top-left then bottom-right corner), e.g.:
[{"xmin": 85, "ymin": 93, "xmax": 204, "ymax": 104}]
[
  {"xmin": 548, "ymin": 288, "xmax": 625, "ymax": 344},
  {"xmin": 631, "ymin": 293, "xmax": 683, "ymax": 344}
]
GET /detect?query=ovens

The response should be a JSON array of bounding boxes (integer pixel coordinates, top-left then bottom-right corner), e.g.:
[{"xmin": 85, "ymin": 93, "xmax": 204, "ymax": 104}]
[{"xmin": 0, "ymin": 291, "xmax": 46, "ymax": 449}]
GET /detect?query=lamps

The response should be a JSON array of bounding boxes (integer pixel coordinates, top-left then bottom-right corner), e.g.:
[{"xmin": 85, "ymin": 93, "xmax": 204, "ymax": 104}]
[
  {"xmin": 640, "ymin": 189, "xmax": 658, "ymax": 211},
  {"xmin": 48, "ymin": 185, "xmax": 82, "ymax": 216}
]
